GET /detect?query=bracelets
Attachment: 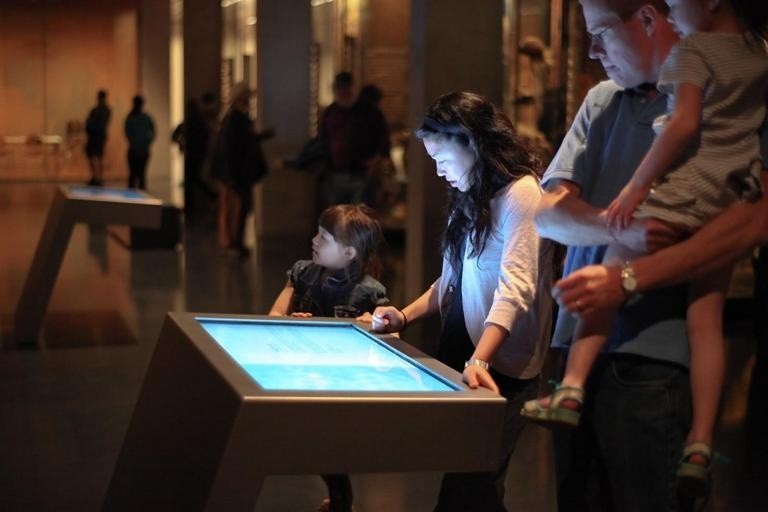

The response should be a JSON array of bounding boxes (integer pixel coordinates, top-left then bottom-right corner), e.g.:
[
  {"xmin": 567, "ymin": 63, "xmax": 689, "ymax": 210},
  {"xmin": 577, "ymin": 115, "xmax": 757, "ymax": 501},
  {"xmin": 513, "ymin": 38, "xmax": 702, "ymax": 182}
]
[
  {"xmin": 399, "ymin": 310, "xmax": 409, "ymax": 328},
  {"xmin": 462, "ymin": 358, "xmax": 491, "ymax": 372}
]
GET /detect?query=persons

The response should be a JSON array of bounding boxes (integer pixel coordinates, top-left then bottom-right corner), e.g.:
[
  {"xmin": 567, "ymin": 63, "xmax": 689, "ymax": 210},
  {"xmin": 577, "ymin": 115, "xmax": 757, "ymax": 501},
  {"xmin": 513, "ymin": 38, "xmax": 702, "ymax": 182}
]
[
  {"xmin": 86, "ymin": 89, "xmax": 111, "ymax": 187},
  {"xmin": 172, "ymin": 81, "xmax": 272, "ymax": 260},
  {"xmin": 125, "ymin": 96, "xmax": 155, "ymax": 189},
  {"xmin": 268, "ymin": 201, "xmax": 404, "ymax": 512},
  {"xmin": 372, "ymin": 90, "xmax": 556, "ymax": 510},
  {"xmin": 315, "ymin": 70, "xmax": 409, "ymax": 307}
]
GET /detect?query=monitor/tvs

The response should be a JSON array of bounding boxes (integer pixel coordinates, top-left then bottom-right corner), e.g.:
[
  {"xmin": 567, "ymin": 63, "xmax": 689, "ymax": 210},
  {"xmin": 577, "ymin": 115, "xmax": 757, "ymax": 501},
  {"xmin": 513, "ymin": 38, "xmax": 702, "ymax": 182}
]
[
  {"xmin": 68, "ymin": 186, "xmax": 147, "ymax": 199},
  {"xmin": 195, "ymin": 316, "xmax": 463, "ymax": 391}
]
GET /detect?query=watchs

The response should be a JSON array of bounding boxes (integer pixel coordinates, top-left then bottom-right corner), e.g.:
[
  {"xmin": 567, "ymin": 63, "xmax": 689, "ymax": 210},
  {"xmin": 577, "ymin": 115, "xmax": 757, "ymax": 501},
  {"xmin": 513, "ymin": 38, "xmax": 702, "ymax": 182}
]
[{"xmin": 617, "ymin": 258, "xmax": 638, "ymax": 299}]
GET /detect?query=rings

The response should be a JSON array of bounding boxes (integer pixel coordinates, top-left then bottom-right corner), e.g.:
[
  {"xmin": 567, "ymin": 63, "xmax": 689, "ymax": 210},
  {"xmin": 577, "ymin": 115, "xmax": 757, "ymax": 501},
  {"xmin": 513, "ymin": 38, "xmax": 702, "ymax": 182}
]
[{"xmin": 575, "ymin": 299, "xmax": 582, "ymax": 311}]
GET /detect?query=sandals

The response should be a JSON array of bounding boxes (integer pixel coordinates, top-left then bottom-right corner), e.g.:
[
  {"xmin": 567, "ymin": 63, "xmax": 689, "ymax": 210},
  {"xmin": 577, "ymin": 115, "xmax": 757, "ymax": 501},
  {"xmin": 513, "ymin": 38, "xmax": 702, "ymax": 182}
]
[
  {"xmin": 520, "ymin": 386, "xmax": 584, "ymax": 424},
  {"xmin": 676, "ymin": 442, "xmax": 716, "ymax": 479}
]
